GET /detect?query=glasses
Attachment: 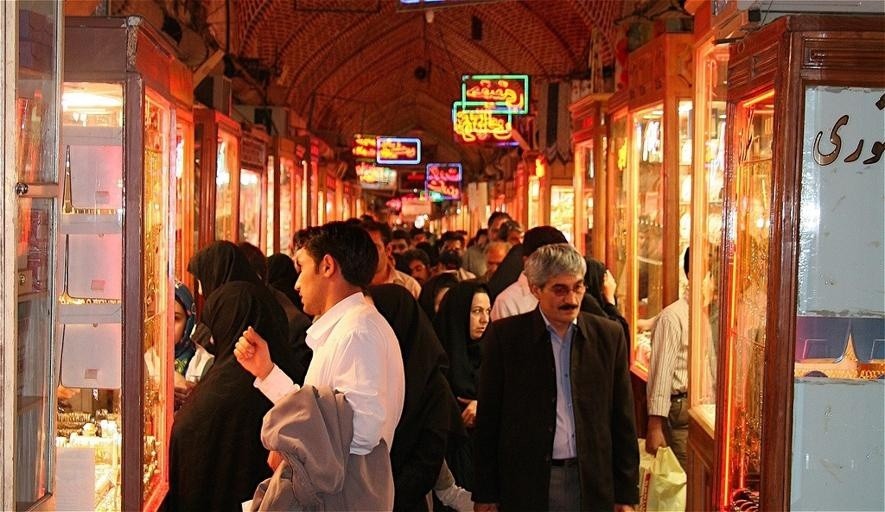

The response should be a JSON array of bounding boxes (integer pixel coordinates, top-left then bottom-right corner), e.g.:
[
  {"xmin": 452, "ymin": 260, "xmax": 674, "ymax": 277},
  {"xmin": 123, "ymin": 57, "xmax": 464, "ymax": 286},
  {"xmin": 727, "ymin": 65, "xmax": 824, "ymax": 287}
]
[{"xmin": 543, "ymin": 283, "xmax": 587, "ymax": 297}]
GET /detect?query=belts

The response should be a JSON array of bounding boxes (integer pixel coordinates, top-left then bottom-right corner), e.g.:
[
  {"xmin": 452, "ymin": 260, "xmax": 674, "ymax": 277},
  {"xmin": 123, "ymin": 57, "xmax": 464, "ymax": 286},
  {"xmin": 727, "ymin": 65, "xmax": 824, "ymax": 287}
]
[{"xmin": 552, "ymin": 457, "xmax": 576, "ymax": 467}]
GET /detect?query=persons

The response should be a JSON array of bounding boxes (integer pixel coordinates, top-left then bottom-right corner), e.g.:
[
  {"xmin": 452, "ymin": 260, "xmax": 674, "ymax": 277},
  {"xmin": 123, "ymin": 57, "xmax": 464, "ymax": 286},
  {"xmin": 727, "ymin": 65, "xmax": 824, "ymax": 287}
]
[{"xmin": 169, "ymin": 210, "xmax": 716, "ymax": 512}]
